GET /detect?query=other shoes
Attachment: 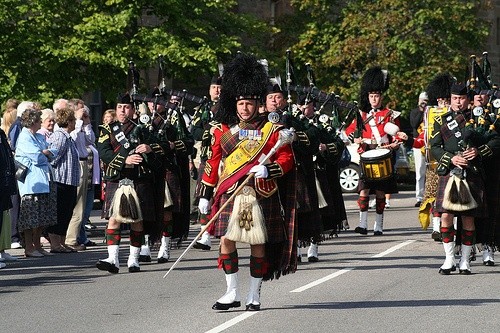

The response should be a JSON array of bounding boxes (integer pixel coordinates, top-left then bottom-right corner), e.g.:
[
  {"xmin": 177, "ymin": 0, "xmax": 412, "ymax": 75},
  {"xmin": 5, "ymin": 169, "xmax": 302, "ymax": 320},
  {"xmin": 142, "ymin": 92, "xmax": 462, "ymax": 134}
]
[
  {"xmin": 368, "ymin": 199, "xmax": 377, "ymax": 209},
  {"xmin": 415, "ymin": 202, "xmax": 421, "ymax": 207},
  {"xmin": 25, "ymin": 240, "xmax": 96, "ymax": 257},
  {"xmin": 385, "ymin": 199, "xmax": 390, "ymax": 208},
  {"xmin": 0, "ymin": 252, "xmax": 18, "ymax": 268},
  {"xmin": 85, "ymin": 222, "xmax": 97, "ymax": 230}
]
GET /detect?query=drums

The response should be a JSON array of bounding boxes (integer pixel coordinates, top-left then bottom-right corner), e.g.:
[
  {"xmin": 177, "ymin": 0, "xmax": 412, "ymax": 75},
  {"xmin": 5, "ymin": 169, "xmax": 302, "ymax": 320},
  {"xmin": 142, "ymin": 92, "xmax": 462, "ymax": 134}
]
[{"xmin": 359, "ymin": 147, "xmax": 394, "ymax": 180}]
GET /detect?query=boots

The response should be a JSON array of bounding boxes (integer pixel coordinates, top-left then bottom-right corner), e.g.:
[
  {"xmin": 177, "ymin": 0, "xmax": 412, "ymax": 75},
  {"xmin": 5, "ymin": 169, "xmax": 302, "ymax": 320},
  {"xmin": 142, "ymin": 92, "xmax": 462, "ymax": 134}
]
[
  {"xmin": 354, "ymin": 211, "xmax": 383, "ymax": 235},
  {"xmin": 432, "ymin": 217, "xmax": 442, "ymax": 242},
  {"xmin": 212, "ymin": 272, "xmax": 263, "ymax": 310},
  {"xmin": 96, "ymin": 245, "xmax": 140, "ymax": 274},
  {"xmin": 296, "ymin": 242, "xmax": 319, "ymax": 263},
  {"xmin": 193, "ymin": 225, "xmax": 211, "ymax": 251},
  {"xmin": 139, "ymin": 235, "xmax": 172, "ymax": 263},
  {"xmin": 438, "ymin": 242, "xmax": 494, "ymax": 275}
]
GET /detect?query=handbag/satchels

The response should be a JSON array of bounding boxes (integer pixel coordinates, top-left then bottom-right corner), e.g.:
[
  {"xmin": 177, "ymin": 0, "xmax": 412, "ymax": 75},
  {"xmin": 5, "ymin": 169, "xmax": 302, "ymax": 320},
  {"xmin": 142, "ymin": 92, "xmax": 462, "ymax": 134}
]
[{"xmin": 12, "ymin": 148, "xmax": 33, "ymax": 183}]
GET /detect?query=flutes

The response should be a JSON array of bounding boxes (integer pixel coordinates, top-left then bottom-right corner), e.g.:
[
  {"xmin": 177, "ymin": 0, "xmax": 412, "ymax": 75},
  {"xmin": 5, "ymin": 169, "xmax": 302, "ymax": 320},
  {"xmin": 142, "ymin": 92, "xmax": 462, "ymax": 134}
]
[
  {"xmin": 458, "ymin": 51, "xmax": 500, "ymax": 181},
  {"xmin": 96, "ymin": 52, "xmax": 209, "ymax": 170},
  {"xmin": 283, "ymin": 50, "xmax": 357, "ymax": 139}
]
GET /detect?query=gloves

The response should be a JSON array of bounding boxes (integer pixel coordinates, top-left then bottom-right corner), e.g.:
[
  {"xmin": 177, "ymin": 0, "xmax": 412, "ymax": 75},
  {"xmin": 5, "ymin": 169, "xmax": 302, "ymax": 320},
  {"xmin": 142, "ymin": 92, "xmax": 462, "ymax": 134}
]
[
  {"xmin": 250, "ymin": 166, "xmax": 269, "ymax": 180},
  {"xmin": 198, "ymin": 198, "xmax": 209, "ymax": 215}
]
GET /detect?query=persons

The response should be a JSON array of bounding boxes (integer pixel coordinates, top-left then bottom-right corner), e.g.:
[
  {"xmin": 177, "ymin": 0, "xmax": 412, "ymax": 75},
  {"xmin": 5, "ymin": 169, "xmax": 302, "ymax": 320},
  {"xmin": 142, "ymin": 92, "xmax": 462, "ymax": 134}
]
[
  {"xmin": 197, "ymin": 87, "xmax": 294, "ymax": 312},
  {"xmin": 346, "ymin": 85, "xmax": 408, "ymax": 236},
  {"xmin": 96, "ymin": 72, "xmax": 231, "ymax": 274},
  {"xmin": 0, "ymin": 98, "xmax": 102, "ymax": 268},
  {"xmin": 258, "ymin": 92, "xmax": 350, "ymax": 262},
  {"xmin": 410, "ymin": 80, "xmax": 500, "ymax": 275}
]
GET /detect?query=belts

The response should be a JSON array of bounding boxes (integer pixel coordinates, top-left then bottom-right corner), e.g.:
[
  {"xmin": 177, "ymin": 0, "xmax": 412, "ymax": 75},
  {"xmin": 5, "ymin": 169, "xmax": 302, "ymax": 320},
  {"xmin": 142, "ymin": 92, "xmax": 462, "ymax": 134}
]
[{"xmin": 79, "ymin": 157, "xmax": 88, "ymax": 161}]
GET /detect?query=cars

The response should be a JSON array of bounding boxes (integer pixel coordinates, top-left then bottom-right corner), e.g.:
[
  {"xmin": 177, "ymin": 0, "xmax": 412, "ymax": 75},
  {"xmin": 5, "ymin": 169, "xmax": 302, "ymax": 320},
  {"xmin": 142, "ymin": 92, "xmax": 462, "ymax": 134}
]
[{"xmin": 338, "ymin": 130, "xmax": 416, "ymax": 193}]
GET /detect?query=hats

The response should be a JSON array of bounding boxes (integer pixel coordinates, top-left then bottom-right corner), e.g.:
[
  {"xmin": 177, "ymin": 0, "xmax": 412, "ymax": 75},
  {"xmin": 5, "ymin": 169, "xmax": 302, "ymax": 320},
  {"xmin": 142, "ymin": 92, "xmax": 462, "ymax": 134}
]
[
  {"xmin": 419, "ymin": 92, "xmax": 428, "ymax": 98},
  {"xmin": 235, "ymin": 84, "xmax": 261, "ymax": 101},
  {"xmin": 210, "ymin": 76, "xmax": 221, "ymax": 86},
  {"xmin": 116, "ymin": 93, "xmax": 134, "ymax": 104},
  {"xmin": 437, "ymin": 92, "xmax": 451, "ymax": 101},
  {"xmin": 367, "ymin": 85, "xmax": 383, "ymax": 94},
  {"xmin": 451, "ymin": 84, "xmax": 466, "ymax": 96},
  {"xmin": 145, "ymin": 87, "xmax": 165, "ymax": 104}
]
